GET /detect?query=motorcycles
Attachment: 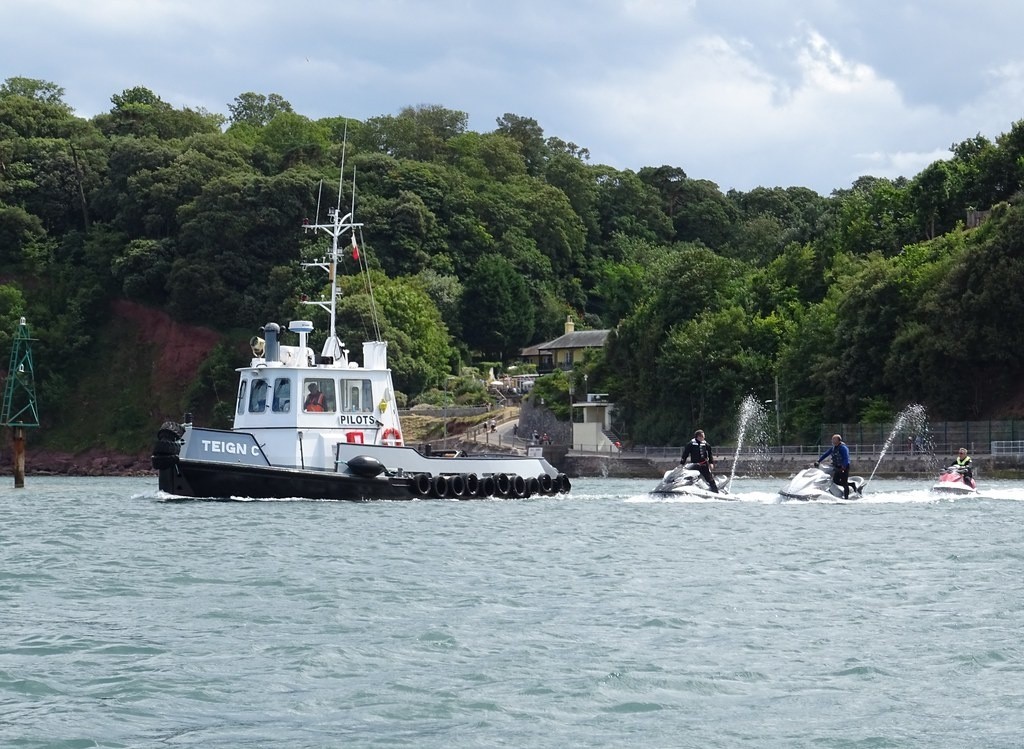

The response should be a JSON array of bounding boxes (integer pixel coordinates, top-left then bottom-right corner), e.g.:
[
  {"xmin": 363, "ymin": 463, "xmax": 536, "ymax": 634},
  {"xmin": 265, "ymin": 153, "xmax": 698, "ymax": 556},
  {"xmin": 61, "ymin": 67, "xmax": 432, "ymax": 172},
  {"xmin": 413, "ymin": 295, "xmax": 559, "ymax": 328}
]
[
  {"xmin": 777, "ymin": 460, "xmax": 867, "ymax": 502},
  {"xmin": 931, "ymin": 463, "xmax": 977, "ymax": 494},
  {"xmin": 651, "ymin": 459, "xmax": 735, "ymax": 501}
]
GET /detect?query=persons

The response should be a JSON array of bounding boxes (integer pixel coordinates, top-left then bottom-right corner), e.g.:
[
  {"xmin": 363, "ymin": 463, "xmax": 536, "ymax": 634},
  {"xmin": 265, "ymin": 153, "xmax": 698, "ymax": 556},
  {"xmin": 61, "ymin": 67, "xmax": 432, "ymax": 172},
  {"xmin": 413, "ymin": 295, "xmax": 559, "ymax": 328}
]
[
  {"xmin": 951, "ymin": 448, "xmax": 972, "ymax": 488},
  {"xmin": 482, "ymin": 417, "xmax": 552, "ymax": 445},
  {"xmin": 679, "ymin": 429, "xmax": 719, "ymax": 493},
  {"xmin": 816, "ymin": 434, "xmax": 857, "ymax": 500},
  {"xmin": 304, "ymin": 383, "xmax": 328, "ymax": 412}
]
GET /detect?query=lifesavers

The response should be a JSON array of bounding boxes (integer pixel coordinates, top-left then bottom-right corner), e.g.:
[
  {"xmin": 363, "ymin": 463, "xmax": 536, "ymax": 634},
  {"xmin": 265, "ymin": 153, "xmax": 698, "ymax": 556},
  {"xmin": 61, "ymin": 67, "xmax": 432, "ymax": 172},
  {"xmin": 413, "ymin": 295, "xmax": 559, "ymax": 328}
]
[{"xmin": 383, "ymin": 428, "xmax": 402, "ymax": 448}]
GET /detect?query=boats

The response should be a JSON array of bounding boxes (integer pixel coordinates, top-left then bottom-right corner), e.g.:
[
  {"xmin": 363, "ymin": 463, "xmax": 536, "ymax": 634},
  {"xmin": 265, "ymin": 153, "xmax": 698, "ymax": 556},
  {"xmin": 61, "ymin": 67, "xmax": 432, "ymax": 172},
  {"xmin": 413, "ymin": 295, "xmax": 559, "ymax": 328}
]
[{"xmin": 153, "ymin": 119, "xmax": 572, "ymax": 502}]
